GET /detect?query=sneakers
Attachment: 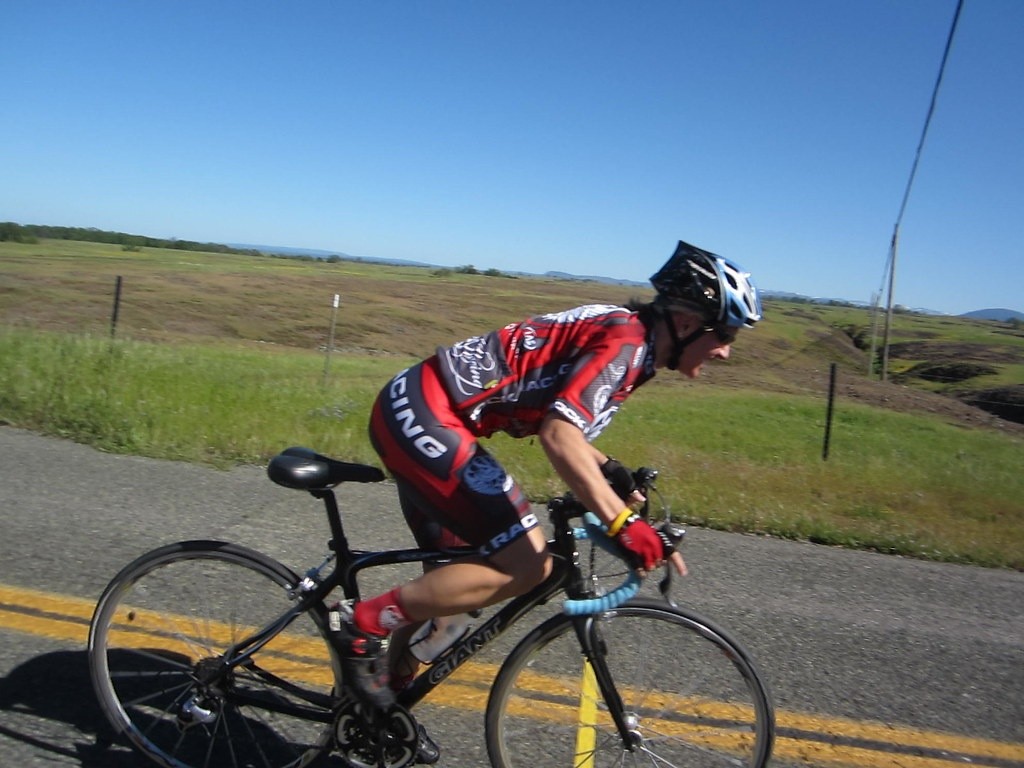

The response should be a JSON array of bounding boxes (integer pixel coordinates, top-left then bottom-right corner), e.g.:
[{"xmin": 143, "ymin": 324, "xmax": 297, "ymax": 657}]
[
  {"xmin": 416, "ymin": 724, "xmax": 442, "ymax": 765},
  {"xmin": 322, "ymin": 598, "xmax": 396, "ymax": 706}
]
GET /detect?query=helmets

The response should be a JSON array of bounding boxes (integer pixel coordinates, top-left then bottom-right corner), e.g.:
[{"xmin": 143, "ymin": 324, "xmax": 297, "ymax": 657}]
[{"xmin": 649, "ymin": 239, "xmax": 762, "ymax": 328}]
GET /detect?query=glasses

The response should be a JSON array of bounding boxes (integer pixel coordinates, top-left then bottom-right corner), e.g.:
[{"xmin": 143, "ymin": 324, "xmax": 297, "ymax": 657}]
[{"xmin": 715, "ymin": 326, "xmax": 736, "ymax": 345}]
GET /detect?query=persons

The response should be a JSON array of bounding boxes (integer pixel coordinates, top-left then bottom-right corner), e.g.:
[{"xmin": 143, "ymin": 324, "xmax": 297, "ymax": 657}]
[{"xmin": 325, "ymin": 240, "xmax": 763, "ymax": 764}]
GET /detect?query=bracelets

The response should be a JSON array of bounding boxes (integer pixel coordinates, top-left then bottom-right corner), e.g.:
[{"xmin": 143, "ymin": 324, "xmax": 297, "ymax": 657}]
[{"xmin": 607, "ymin": 508, "xmax": 633, "ymax": 537}]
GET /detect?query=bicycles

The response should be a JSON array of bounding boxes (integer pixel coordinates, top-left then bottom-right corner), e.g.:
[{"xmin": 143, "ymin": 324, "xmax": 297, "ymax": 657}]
[{"xmin": 85, "ymin": 443, "xmax": 781, "ymax": 768}]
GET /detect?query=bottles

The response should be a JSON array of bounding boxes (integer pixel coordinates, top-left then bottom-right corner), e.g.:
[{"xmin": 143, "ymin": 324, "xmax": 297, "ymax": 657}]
[{"xmin": 409, "ymin": 608, "xmax": 482, "ymax": 662}]
